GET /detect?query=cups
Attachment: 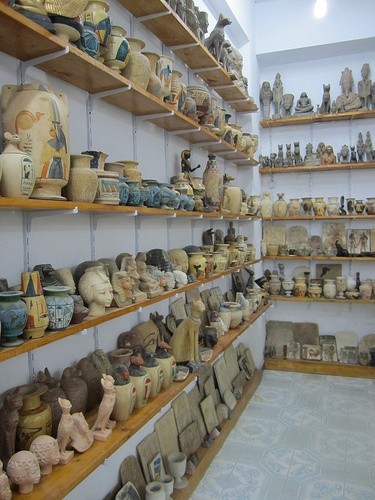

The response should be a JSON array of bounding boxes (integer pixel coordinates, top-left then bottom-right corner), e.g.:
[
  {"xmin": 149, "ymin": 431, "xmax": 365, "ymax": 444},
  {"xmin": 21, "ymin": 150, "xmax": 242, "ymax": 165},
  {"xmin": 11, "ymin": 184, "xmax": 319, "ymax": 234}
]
[{"xmin": 146, "ymin": 481, "xmax": 165, "ymax": 500}]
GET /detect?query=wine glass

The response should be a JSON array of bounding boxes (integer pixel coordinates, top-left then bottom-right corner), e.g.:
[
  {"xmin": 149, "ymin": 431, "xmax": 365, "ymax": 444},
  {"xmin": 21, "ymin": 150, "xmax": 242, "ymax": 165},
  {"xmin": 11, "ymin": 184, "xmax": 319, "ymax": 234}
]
[
  {"xmin": 346, "ymin": 198, "xmax": 355, "ymax": 216},
  {"xmin": 335, "ymin": 276, "xmax": 347, "ymax": 299},
  {"xmin": 159, "ymin": 475, "xmax": 174, "ymax": 500},
  {"xmin": 168, "ymin": 453, "xmax": 189, "ymax": 489}
]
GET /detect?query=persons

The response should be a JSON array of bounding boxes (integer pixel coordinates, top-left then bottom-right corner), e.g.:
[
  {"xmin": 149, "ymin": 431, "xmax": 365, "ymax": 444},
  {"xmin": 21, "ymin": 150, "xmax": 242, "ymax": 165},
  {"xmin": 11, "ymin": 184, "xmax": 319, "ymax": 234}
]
[
  {"xmin": 78, "ymin": 267, "xmax": 114, "ymax": 316},
  {"xmin": 294, "ymin": 92, "xmax": 314, "ymax": 112},
  {"xmin": 112, "ymin": 249, "xmax": 188, "ymax": 300},
  {"xmin": 0, "ymin": 434, "xmax": 60, "ymax": 500},
  {"xmin": 181, "ymin": 150, "xmax": 202, "ymax": 190}
]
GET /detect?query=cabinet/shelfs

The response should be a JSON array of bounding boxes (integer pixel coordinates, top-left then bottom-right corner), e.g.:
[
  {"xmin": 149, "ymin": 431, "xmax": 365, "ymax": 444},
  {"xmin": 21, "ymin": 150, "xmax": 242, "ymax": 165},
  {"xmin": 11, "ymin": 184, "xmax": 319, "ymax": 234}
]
[
  {"xmin": 258, "ymin": 66, "xmax": 375, "ymax": 380},
  {"xmin": 0, "ymin": 0, "xmax": 274, "ymax": 500}
]
